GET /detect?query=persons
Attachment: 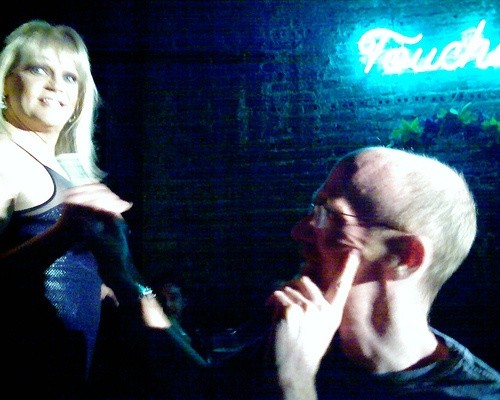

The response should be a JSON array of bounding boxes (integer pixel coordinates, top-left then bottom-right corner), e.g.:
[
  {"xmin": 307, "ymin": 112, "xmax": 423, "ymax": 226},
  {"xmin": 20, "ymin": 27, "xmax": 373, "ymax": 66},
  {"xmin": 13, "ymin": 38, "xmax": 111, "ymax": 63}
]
[
  {"xmin": 0, "ymin": 21, "xmax": 132, "ymax": 400},
  {"xmin": 89, "ymin": 146, "xmax": 499, "ymax": 400}
]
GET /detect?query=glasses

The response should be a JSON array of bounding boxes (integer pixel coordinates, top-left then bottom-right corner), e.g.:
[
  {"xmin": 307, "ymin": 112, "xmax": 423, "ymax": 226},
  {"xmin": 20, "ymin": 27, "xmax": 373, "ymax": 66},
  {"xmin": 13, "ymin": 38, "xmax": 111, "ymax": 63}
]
[{"xmin": 302, "ymin": 202, "xmax": 371, "ymax": 230}]
